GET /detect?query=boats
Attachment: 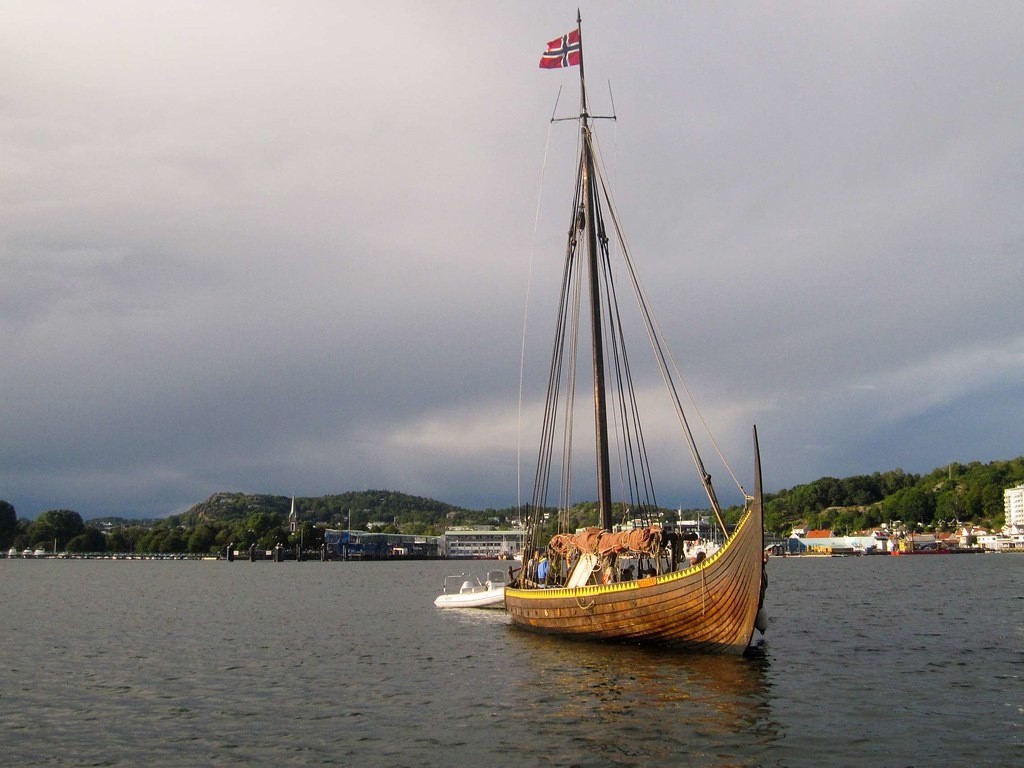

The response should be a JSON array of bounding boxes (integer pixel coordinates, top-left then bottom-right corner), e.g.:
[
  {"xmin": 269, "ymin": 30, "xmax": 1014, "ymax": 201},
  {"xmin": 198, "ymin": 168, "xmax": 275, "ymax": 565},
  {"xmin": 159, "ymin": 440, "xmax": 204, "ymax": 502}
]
[
  {"xmin": 8, "ymin": 548, "xmax": 17, "ymax": 559},
  {"xmin": 22, "ymin": 549, "xmax": 32, "ymax": 559},
  {"xmin": 434, "ymin": 569, "xmax": 506, "ymax": 609},
  {"xmin": 33, "ymin": 548, "xmax": 46, "ymax": 559}
]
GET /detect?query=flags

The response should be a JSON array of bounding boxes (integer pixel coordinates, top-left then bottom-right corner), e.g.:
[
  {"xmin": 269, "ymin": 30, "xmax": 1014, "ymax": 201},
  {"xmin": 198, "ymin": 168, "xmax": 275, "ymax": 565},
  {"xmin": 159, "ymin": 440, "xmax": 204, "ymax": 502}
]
[{"xmin": 539, "ymin": 29, "xmax": 582, "ymax": 69}]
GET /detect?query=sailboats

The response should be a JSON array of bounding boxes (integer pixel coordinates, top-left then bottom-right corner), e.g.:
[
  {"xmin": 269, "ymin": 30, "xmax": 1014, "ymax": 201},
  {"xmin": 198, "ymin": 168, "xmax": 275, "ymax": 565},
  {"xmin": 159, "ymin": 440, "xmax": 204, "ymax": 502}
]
[{"xmin": 504, "ymin": 8, "xmax": 767, "ymax": 659}]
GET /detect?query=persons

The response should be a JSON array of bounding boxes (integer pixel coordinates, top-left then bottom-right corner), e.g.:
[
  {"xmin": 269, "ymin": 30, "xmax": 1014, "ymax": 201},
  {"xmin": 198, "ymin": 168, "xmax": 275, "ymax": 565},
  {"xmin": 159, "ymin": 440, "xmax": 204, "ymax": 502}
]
[{"xmin": 528, "ymin": 550, "xmax": 560, "ymax": 584}]
